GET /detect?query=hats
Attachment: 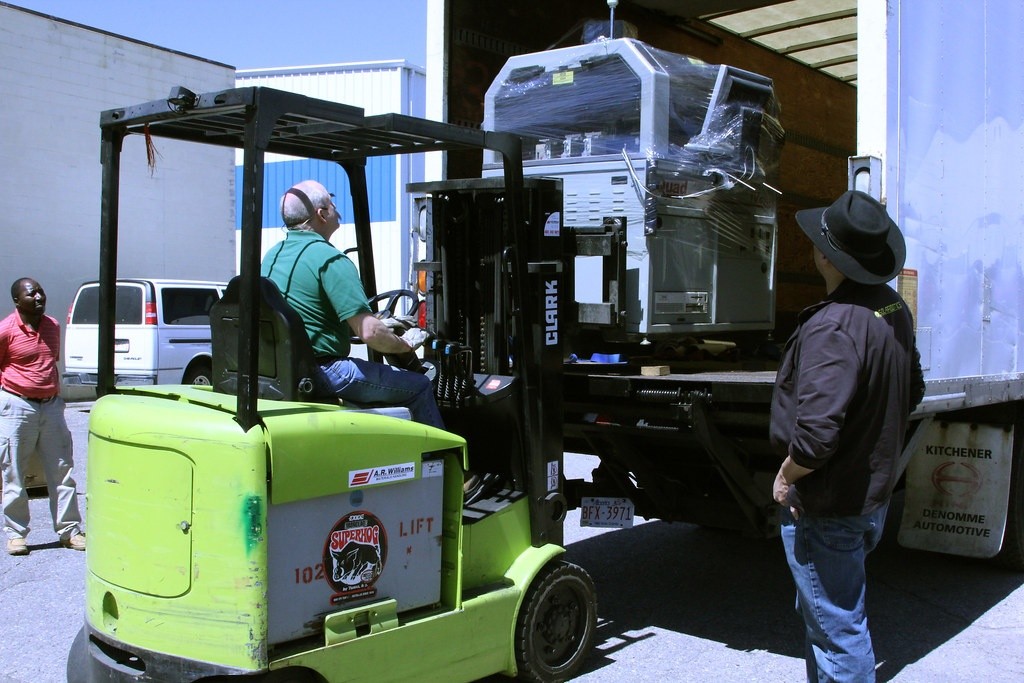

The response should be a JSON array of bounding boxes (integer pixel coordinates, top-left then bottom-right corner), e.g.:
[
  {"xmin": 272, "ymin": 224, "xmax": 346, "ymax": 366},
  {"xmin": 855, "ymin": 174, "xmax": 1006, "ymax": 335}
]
[{"xmin": 795, "ymin": 190, "xmax": 907, "ymax": 285}]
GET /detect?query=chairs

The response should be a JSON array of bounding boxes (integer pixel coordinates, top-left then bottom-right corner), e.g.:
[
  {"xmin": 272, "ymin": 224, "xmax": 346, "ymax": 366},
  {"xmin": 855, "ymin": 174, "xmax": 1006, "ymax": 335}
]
[{"xmin": 208, "ymin": 276, "xmax": 414, "ymax": 420}]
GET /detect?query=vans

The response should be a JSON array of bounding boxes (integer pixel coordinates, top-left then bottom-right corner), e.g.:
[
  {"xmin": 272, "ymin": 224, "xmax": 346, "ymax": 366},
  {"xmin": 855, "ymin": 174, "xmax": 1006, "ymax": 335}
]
[{"xmin": 62, "ymin": 279, "xmax": 228, "ymax": 393}]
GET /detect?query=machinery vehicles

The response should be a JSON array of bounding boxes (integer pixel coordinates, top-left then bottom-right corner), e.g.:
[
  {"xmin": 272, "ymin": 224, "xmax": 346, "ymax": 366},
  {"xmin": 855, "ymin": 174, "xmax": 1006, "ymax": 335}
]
[{"xmin": 84, "ymin": 84, "xmax": 598, "ymax": 683}]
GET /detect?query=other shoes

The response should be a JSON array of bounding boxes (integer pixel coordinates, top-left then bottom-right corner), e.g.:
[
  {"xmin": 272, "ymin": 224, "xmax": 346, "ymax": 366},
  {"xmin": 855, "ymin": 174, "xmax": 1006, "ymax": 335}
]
[{"xmin": 464, "ymin": 474, "xmax": 481, "ymax": 499}]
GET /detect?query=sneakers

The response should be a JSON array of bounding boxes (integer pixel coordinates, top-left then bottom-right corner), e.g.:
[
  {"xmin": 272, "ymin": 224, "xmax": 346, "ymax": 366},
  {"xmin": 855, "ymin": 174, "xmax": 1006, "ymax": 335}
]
[
  {"xmin": 59, "ymin": 532, "xmax": 86, "ymax": 550},
  {"xmin": 7, "ymin": 538, "xmax": 29, "ymax": 554}
]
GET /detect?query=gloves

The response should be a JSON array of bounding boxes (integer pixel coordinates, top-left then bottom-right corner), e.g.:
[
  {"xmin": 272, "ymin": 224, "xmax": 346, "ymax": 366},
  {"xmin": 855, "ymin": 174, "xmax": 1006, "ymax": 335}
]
[
  {"xmin": 402, "ymin": 328, "xmax": 430, "ymax": 349},
  {"xmin": 380, "ymin": 318, "xmax": 405, "ymax": 329}
]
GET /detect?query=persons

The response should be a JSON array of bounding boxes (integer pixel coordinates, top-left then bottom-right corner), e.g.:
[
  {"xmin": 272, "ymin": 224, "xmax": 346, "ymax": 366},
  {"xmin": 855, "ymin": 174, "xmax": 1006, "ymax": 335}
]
[
  {"xmin": 770, "ymin": 190, "xmax": 927, "ymax": 682},
  {"xmin": 1, "ymin": 278, "xmax": 89, "ymax": 554},
  {"xmin": 262, "ymin": 181, "xmax": 482, "ymax": 494}
]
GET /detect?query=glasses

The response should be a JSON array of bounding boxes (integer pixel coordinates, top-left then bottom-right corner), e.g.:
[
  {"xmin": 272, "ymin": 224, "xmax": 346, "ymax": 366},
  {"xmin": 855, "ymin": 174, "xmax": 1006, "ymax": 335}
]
[{"xmin": 281, "ymin": 211, "xmax": 317, "ymax": 232}]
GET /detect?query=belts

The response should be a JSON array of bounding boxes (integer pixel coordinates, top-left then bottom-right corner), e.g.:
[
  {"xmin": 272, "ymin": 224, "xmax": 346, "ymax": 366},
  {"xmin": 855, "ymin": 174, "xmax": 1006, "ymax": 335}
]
[
  {"xmin": 317, "ymin": 355, "xmax": 335, "ymax": 367},
  {"xmin": 2, "ymin": 387, "xmax": 57, "ymax": 404}
]
